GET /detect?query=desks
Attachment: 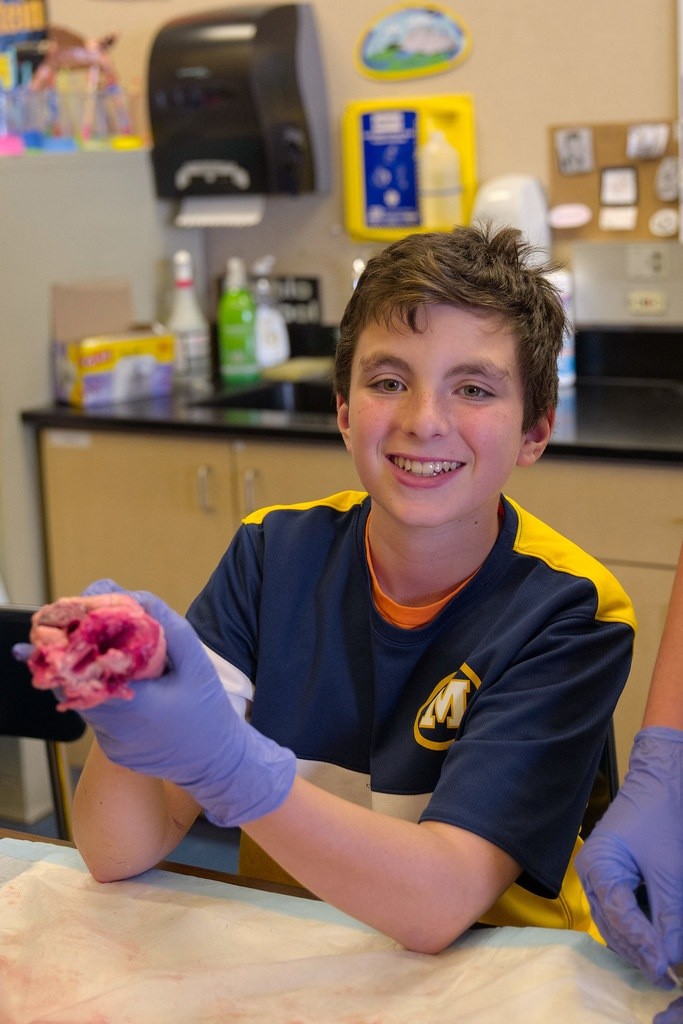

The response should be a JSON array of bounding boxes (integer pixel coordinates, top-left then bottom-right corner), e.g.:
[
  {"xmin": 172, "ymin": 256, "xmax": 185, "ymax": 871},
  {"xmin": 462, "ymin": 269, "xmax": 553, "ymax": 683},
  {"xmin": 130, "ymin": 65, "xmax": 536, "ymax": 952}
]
[{"xmin": 1, "ymin": 827, "xmax": 682, "ymax": 1024}]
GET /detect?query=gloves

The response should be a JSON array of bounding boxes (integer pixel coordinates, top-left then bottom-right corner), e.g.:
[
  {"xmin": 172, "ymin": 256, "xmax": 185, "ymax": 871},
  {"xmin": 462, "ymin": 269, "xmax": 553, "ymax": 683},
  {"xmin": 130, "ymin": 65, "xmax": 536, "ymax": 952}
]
[
  {"xmin": 574, "ymin": 726, "xmax": 683, "ymax": 990},
  {"xmin": 653, "ymin": 997, "xmax": 683, "ymax": 1024},
  {"xmin": 13, "ymin": 579, "xmax": 296, "ymax": 826}
]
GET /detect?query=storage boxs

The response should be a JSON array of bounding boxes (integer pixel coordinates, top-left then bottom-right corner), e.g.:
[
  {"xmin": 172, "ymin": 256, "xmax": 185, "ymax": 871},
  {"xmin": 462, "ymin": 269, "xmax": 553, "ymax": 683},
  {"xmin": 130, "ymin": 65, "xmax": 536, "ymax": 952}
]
[{"xmin": 50, "ymin": 284, "xmax": 176, "ymax": 409}]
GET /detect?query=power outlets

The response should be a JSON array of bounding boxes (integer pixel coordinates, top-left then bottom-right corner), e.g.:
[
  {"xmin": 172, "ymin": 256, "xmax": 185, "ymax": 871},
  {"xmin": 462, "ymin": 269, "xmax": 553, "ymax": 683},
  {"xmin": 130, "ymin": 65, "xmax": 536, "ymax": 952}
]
[{"xmin": 627, "ymin": 245, "xmax": 670, "ymax": 280}]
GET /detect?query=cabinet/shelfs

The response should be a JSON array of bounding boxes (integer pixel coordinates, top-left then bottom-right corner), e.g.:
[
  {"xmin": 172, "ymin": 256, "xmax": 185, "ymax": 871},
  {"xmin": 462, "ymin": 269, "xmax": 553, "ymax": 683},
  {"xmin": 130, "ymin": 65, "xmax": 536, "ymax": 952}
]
[{"xmin": 20, "ymin": 410, "xmax": 683, "ymax": 784}]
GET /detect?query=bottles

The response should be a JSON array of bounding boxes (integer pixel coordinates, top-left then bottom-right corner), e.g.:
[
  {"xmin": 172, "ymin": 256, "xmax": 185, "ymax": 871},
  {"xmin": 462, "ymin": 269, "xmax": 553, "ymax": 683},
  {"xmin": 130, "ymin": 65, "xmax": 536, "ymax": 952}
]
[
  {"xmin": 218, "ymin": 256, "xmax": 259, "ymax": 375},
  {"xmin": 168, "ymin": 251, "xmax": 210, "ymax": 372}
]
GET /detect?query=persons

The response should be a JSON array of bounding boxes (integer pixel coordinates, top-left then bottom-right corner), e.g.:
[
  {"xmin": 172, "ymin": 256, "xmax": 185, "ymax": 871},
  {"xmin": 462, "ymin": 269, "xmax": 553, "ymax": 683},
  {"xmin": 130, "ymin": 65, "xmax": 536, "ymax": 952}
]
[
  {"xmin": 17, "ymin": 222, "xmax": 640, "ymax": 955},
  {"xmin": 578, "ymin": 547, "xmax": 682, "ymax": 986}
]
